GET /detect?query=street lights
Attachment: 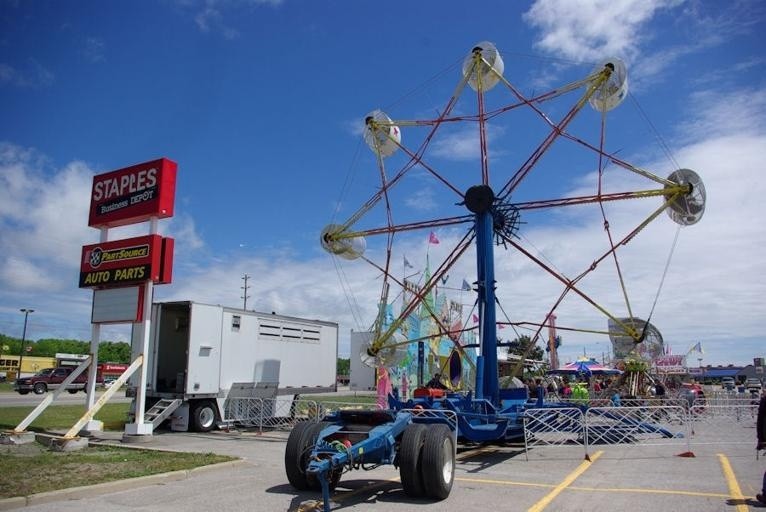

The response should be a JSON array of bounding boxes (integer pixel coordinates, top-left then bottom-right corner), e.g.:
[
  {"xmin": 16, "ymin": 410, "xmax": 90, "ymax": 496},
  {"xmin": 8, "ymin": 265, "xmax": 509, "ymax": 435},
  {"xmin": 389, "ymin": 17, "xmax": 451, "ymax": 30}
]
[{"xmin": 18, "ymin": 307, "xmax": 35, "ymax": 379}]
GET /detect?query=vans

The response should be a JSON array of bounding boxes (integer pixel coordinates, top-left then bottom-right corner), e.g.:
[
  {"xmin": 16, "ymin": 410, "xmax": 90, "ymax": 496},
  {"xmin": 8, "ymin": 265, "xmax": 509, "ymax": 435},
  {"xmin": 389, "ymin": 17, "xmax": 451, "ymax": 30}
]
[{"xmin": 13, "ymin": 366, "xmax": 105, "ymax": 394}]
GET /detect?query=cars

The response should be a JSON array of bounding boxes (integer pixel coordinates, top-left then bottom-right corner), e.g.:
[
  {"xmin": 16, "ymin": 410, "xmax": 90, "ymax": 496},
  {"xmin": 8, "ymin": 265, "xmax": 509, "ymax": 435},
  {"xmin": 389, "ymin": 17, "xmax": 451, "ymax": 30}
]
[
  {"xmin": 743, "ymin": 377, "xmax": 762, "ymax": 390},
  {"xmin": 721, "ymin": 376, "xmax": 736, "ymax": 389}
]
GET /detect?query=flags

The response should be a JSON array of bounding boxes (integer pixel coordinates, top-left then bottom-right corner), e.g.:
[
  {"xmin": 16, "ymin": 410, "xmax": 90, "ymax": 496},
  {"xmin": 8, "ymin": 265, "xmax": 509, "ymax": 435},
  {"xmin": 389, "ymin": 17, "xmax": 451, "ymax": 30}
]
[
  {"xmin": 404, "ymin": 255, "xmax": 411, "ymax": 267},
  {"xmin": 430, "ymin": 230, "xmax": 439, "ymax": 244},
  {"xmin": 461, "ymin": 279, "xmax": 471, "ymax": 290}
]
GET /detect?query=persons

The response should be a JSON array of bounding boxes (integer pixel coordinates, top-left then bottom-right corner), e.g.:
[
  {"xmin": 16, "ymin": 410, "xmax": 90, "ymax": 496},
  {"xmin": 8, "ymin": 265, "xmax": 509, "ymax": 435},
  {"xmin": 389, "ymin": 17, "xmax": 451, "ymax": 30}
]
[
  {"xmin": 755, "ymin": 388, "xmax": 766, "ymax": 503},
  {"xmin": 523, "ymin": 374, "xmax": 707, "ymax": 426},
  {"xmin": 425, "ymin": 373, "xmax": 447, "ymax": 390}
]
